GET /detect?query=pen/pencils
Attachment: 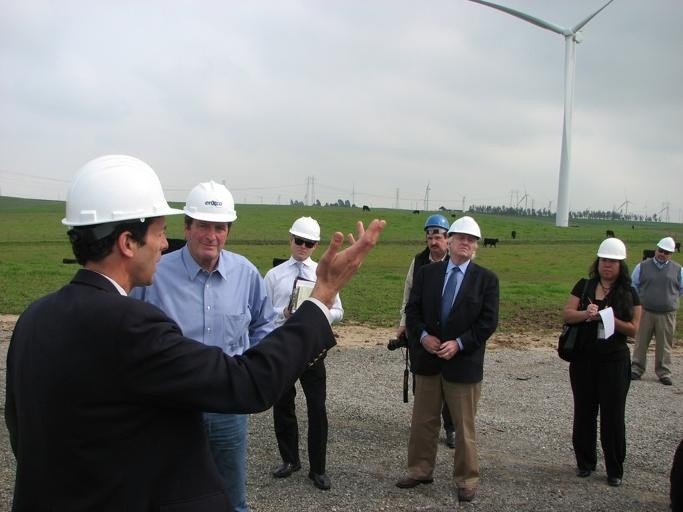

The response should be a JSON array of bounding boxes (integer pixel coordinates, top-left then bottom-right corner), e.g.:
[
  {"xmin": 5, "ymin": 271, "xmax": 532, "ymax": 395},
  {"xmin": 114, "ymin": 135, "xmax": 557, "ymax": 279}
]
[{"xmin": 587, "ymin": 294, "xmax": 596, "ymax": 305}]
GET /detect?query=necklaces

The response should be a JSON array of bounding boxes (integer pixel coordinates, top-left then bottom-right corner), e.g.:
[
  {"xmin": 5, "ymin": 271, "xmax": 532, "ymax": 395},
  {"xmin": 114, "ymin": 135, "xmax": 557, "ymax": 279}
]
[{"xmin": 598, "ymin": 278, "xmax": 614, "ymax": 296}]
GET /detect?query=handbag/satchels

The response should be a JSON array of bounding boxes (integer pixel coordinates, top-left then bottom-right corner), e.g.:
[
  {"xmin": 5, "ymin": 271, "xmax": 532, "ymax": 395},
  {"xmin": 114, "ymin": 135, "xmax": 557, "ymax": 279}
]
[{"xmin": 559, "ymin": 279, "xmax": 589, "ymax": 359}]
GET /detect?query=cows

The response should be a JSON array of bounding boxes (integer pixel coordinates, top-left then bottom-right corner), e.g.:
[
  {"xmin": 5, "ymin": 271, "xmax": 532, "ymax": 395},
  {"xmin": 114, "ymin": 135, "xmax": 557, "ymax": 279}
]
[
  {"xmin": 411, "ymin": 209, "xmax": 420, "ymax": 215},
  {"xmin": 362, "ymin": 205, "xmax": 370, "ymax": 213},
  {"xmin": 511, "ymin": 230, "xmax": 516, "ymax": 240},
  {"xmin": 483, "ymin": 237, "xmax": 499, "ymax": 248},
  {"xmin": 606, "ymin": 230, "xmax": 614, "ymax": 237},
  {"xmin": 643, "ymin": 249, "xmax": 655, "ymax": 261}
]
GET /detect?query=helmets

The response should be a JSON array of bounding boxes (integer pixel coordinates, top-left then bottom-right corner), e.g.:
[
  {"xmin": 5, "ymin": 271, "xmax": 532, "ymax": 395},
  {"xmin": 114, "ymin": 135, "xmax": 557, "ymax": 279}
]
[
  {"xmin": 595, "ymin": 238, "xmax": 627, "ymax": 262},
  {"xmin": 61, "ymin": 154, "xmax": 185, "ymax": 229},
  {"xmin": 181, "ymin": 182, "xmax": 240, "ymax": 221},
  {"xmin": 421, "ymin": 216, "xmax": 446, "ymax": 230},
  {"xmin": 289, "ymin": 218, "xmax": 322, "ymax": 244},
  {"xmin": 449, "ymin": 217, "xmax": 483, "ymax": 236},
  {"xmin": 659, "ymin": 238, "xmax": 675, "ymax": 249}
]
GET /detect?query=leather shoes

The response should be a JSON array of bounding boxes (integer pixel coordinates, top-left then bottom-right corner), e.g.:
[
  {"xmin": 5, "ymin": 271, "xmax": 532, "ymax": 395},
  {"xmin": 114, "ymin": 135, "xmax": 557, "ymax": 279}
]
[
  {"xmin": 396, "ymin": 476, "xmax": 431, "ymax": 489},
  {"xmin": 445, "ymin": 426, "xmax": 452, "ymax": 448},
  {"xmin": 273, "ymin": 462, "xmax": 301, "ymax": 478},
  {"xmin": 576, "ymin": 462, "xmax": 597, "ymax": 479},
  {"xmin": 607, "ymin": 465, "xmax": 624, "ymax": 485},
  {"xmin": 457, "ymin": 485, "xmax": 474, "ymax": 500},
  {"xmin": 661, "ymin": 376, "xmax": 670, "ymax": 384},
  {"xmin": 309, "ymin": 472, "xmax": 328, "ymax": 491},
  {"xmin": 628, "ymin": 374, "xmax": 638, "ymax": 382}
]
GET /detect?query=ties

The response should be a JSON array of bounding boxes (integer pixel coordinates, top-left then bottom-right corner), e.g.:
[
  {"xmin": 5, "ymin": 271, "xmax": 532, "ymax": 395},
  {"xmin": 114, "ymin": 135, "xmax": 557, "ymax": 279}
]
[{"xmin": 436, "ymin": 266, "xmax": 459, "ymax": 327}]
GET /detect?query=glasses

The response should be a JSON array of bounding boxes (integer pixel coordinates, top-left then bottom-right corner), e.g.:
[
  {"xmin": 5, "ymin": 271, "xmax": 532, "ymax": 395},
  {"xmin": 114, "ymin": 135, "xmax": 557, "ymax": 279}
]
[
  {"xmin": 660, "ymin": 249, "xmax": 672, "ymax": 254},
  {"xmin": 292, "ymin": 238, "xmax": 314, "ymax": 248}
]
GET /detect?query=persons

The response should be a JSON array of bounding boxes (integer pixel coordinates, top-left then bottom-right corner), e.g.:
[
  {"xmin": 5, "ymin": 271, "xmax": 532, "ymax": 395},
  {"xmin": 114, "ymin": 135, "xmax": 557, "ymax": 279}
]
[
  {"xmin": 129, "ymin": 182, "xmax": 273, "ymax": 512},
  {"xmin": 396, "ymin": 216, "xmax": 500, "ymax": 501},
  {"xmin": 630, "ymin": 236, "xmax": 683, "ymax": 383},
  {"xmin": 4, "ymin": 155, "xmax": 386, "ymax": 512},
  {"xmin": 561, "ymin": 238, "xmax": 641, "ymax": 486},
  {"xmin": 394, "ymin": 214, "xmax": 455, "ymax": 448},
  {"xmin": 263, "ymin": 216, "xmax": 344, "ymax": 490}
]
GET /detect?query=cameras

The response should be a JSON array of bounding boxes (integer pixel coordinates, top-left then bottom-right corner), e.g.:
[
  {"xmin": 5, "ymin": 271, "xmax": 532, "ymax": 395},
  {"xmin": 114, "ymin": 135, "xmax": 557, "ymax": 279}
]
[{"xmin": 386, "ymin": 331, "xmax": 409, "ymax": 351}]
